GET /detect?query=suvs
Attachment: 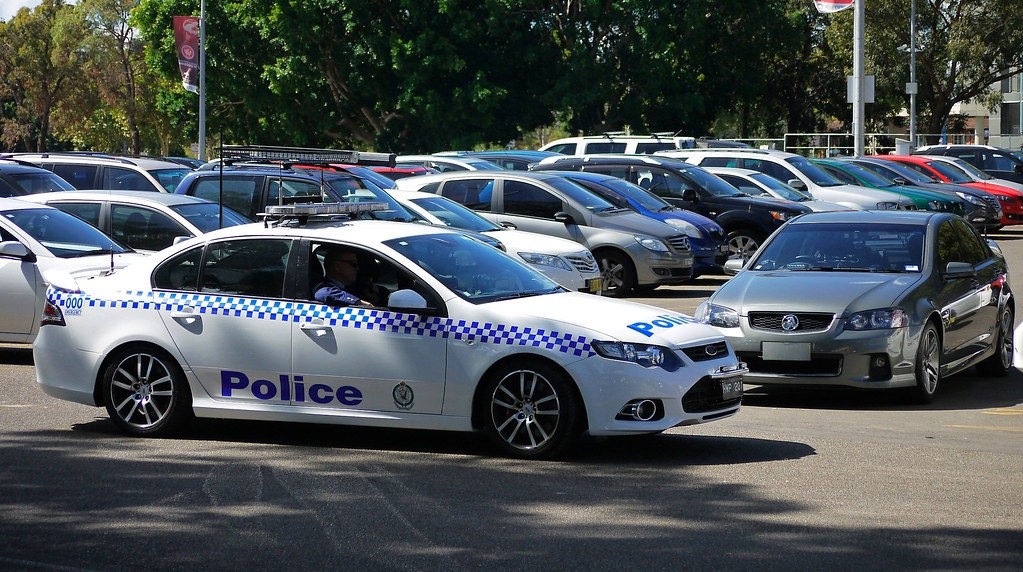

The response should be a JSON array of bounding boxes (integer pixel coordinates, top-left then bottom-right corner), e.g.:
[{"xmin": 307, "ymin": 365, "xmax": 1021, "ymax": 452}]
[{"xmin": 530, "ymin": 154, "xmax": 816, "ymax": 277}]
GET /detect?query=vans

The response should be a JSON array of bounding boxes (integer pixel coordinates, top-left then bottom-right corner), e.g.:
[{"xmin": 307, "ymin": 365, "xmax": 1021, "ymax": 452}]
[{"xmin": 535, "ymin": 132, "xmax": 697, "ymax": 159}]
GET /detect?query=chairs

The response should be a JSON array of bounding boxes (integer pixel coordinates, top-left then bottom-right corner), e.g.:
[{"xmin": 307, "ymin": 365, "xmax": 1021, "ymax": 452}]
[
  {"xmin": 147, "ymin": 214, "xmax": 165, "ymax": 247},
  {"xmin": 640, "ymin": 178, "xmax": 651, "ymax": 189},
  {"xmin": 309, "ymin": 252, "xmax": 324, "ymax": 282},
  {"xmin": 123, "ymin": 212, "xmax": 148, "ymax": 249}
]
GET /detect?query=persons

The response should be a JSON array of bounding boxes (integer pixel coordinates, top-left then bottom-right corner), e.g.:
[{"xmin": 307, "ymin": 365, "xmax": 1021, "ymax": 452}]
[
  {"xmin": 984, "ymin": 128, "xmax": 989, "ymax": 144},
  {"xmin": 897, "ymin": 235, "xmax": 921, "ymax": 270},
  {"xmin": 311, "ymin": 248, "xmax": 381, "ymax": 307}
]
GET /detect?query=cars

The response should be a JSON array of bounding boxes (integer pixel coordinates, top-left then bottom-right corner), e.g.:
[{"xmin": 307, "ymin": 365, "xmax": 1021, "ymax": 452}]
[
  {"xmin": 32, "ymin": 199, "xmax": 751, "ymax": 462},
  {"xmin": 0, "ymin": 150, "xmax": 561, "ymax": 198},
  {"xmin": 7, "ymin": 190, "xmax": 328, "ymax": 277},
  {"xmin": 693, "ymin": 209, "xmax": 1017, "ymax": 407},
  {"xmin": 332, "ymin": 190, "xmax": 605, "ymax": 296},
  {"xmin": 0, "ymin": 157, "xmax": 78, "ymax": 203},
  {"xmin": 1, "ymin": 196, "xmax": 141, "ymax": 360},
  {"xmin": 530, "ymin": 168, "xmax": 731, "ymax": 281},
  {"xmin": 394, "ymin": 169, "xmax": 696, "ymax": 300},
  {"xmin": 651, "ymin": 138, "xmax": 1023, "ymax": 238}
]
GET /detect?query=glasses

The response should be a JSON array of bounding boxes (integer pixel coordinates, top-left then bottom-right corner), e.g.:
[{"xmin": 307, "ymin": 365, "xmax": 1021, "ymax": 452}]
[{"xmin": 336, "ymin": 259, "xmax": 358, "ymax": 267}]
